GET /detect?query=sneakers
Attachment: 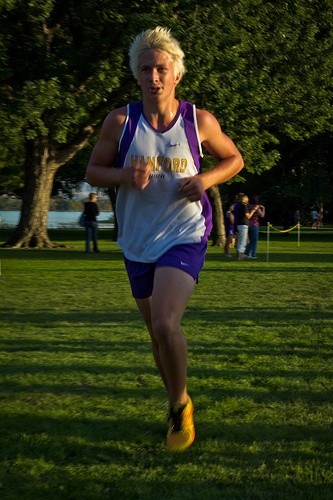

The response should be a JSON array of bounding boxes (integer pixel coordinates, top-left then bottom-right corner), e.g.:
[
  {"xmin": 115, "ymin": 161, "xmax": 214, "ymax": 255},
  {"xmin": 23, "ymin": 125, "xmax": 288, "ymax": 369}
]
[{"xmin": 164, "ymin": 394, "xmax": 195, "ymax": 452}]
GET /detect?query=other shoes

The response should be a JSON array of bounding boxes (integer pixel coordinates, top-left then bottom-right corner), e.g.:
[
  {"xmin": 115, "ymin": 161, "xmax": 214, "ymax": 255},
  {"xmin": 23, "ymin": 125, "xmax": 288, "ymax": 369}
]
[
  {"xmin": 223, "ymin": 254, "xmax": 257, "ymax": 261},
  {"xmin": 85, "ymin": 249, "xmax": 102, "ymax": 254}
]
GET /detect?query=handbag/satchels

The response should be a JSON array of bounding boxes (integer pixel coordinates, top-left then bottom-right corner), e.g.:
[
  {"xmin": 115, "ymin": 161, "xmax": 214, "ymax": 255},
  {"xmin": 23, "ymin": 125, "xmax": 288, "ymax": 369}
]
[{"xmin": 79, "ymin": 213, "xmax": 87, "ymax": 227}]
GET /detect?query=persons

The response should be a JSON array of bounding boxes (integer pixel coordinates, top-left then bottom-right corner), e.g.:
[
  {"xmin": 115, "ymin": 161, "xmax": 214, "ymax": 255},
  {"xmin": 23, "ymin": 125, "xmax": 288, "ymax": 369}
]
[
  {"xmin": 224, "ymin": 192, "xmax": 265, "ymax": 260},
  {"xmin": 311, "ymin": 206, "xmax": 324, "ymax": 229},
  {"xmin": 86, "ymin": 26, "xmax": 244, "ymax": 452},
  {"xmin": 83, "ymin": 193, "xmax": 101, "ymax": 253}
]
